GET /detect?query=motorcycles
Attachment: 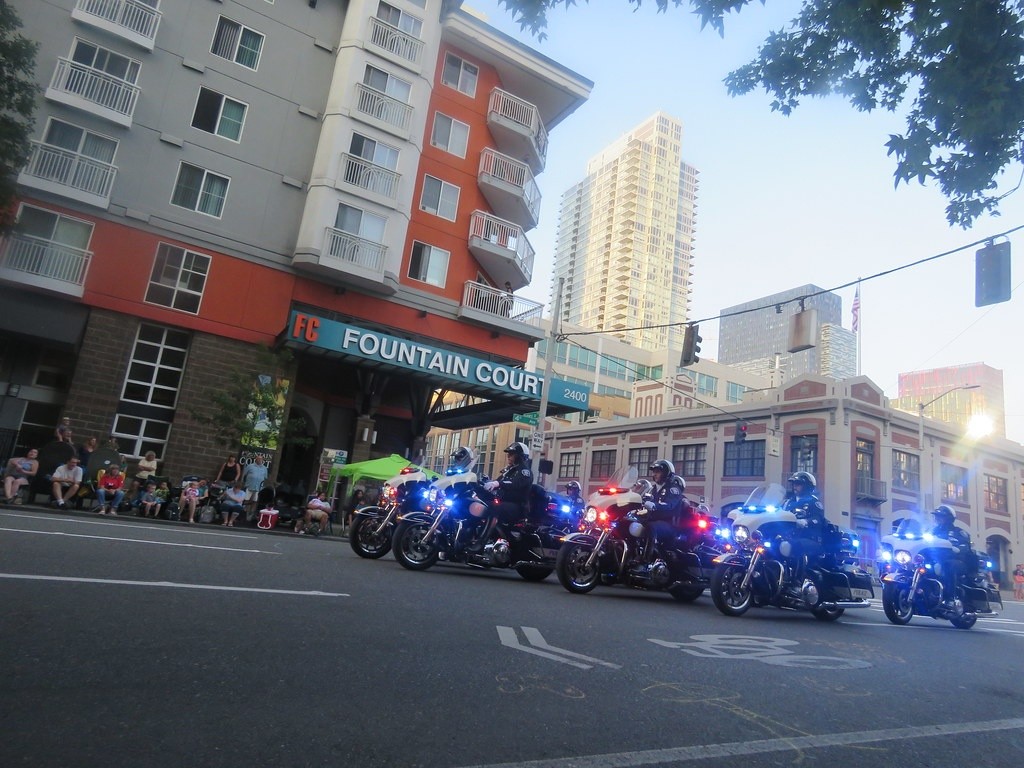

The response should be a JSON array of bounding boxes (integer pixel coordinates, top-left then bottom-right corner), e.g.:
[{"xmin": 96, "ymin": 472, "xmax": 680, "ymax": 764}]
[
  {"xmin": 710, "ymin": 482, "xmax": 876, "ymax": 622},
  {"xmin": 555, "ymin": 464, "xmax": 733, "ymax": 603},
  {"xmin": 349, "ymin": 454, "xmax": 438, "ymax": 557},
  {"xmin": 877, "ymin": 508, "xmax": 1004, "ymax": 630},
  {"xmin": 392, "ymin": 448, "xmax": 575, "ymax": 584}
]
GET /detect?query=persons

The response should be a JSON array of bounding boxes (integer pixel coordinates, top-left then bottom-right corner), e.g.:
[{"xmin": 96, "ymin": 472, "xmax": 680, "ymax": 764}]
[
  {"xmin": 628, "ymin": 459, "xmax": 686, "ymax": 581},
  {"xmin": 1012, "ymin": 563, "xmax": 1024, "ymax": 601},
  {"xmin": 77, "ymin": 436, "xmax": 97, "ymax": 478},
  {"xmin": 220, "ymin": 482, "xmax": 247, "ymax": 527},
  {"xmin": 240, "ymin": 455, "xmax": 269, "ymax": 522},
  {"xmin": 306, "ymin": 491, "xmax": 332, "ymax": 533},
  {"xmin": 778, "ymin": 468, "xmax": 827, "ymax": 588},
  {"xmin": 344, "ymin": 488, "xmax": 367, "ymax": 528},
  {"xmin": 931, "ymin": 505, "xmax": 976, "ymax": 614},
  {"xmin": 177, "ymin": 478, "xmax": 209, "ymax": 524},
  {"xmin": 56, "ymin": 416, "xmax": 75, "ymax": 448},
  {"xmin": 214, "ymin": 454, "xmax": 240, "ymax": 488},
  {"xmin": 565, "ymin": 480, "xmax": 585, "ymax": 523},
  {"xmin": 463, "ymin": 440, "xmax": 533, "ymax": 554},
  {"xmin": 52, "ymin": 456, "xmax": 82, "ymax": 510},
  {"xmin": 141, "ymin": 480, "xmax": 171, "ymax": 520},
  {"xmin": 118, "ymin": 455, "xmax": 128, "ymax": 475},
  {"xmin": 446, "ymin": 446, "xmax": 475, "ymax": 476},
  {"xmin": 134, "ymin": 451, "xmax": 158, "ymax": 485},
  {"xmin": 4, "ymin": 448, "xmax": 42, "ymax": 503},
  {"xmin": 96, "ymin": 463, "xmax": 125, "ymax": 516}
]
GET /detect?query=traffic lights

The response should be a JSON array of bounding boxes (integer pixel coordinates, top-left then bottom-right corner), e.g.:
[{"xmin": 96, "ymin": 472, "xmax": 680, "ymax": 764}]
[
  {"xmin": 734, "ymin": 420, "xmax": 747, "ymax": 446},
  {"xmin": 679, "ymin": 325, "xmax": 704, "ymax": 368}
]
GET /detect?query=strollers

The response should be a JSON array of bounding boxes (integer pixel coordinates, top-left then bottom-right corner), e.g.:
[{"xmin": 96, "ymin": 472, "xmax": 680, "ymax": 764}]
[{"xmin": 206, "ymin": 479, "xmax": 232, "ymax": 525}]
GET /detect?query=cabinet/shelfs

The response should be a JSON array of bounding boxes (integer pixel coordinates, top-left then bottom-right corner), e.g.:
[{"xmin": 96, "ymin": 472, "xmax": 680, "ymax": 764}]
[{"xmin": 316, "ymin": 464, "xmax": 347, "ymax": 512}]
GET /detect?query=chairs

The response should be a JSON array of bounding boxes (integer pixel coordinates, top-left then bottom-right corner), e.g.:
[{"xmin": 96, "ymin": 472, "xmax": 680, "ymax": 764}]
[{"xmin": 0, "ymin": 449, "xmax": 336, "ymax": 534}]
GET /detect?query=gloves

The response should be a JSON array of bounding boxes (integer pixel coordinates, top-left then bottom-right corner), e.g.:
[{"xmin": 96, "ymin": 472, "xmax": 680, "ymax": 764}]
[{"xmin": 484, "ymin": 481, "xmax": 499, "ymax": 492}]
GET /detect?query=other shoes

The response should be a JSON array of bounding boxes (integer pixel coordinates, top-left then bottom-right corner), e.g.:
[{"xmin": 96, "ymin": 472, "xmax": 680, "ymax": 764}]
[
  {"xmin": 98, "ymin": 510, "xmax": 107, "ymax": 514},
  {"xmin": 108, "ymin": 511, "xmax": 117, "ymax": 516},
  {"xmin": 57, "ymin": 500, "xmax": 66, "ymax": 507},
  {"xmin": 14, "ymin": 498, "xmax": 22, "ymax": 504},
  {"xmin": 6, "ymin": 498, "xmax": 14, "ymax": 503}
]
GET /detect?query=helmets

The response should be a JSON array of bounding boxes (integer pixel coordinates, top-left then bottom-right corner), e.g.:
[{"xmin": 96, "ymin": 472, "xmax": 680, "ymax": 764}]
[
  {"xmin": 930, "ymin": 506, "xmax": 955, "ymax": 525},
  {"xmin": 788, "ymin": 471, "xmax": 816, "ymax": 496},
  {"xmin": 503, "ymin": 442, "xmax": 529, "ymax": 460},
  {"xmin": 649, "ymin": 459, "xmax": 675, "ymax": 482},
  {"xmin": 565, "ymin": 481, "xmax": 580, "ymax": 495},
  {"xmin": 673, "ymin": 476, "xmax": 686, "ymax": 495},
  {"xmin": 450, "ymin": 447, "xmax": 473, "ymax": 466}
]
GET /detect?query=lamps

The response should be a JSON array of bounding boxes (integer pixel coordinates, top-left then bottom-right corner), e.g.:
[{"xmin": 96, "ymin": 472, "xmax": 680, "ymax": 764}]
[
  {"xmin": 372, "ymin": 431, "xmax": 377, "ymax": 444},
  {"xmin": 405, "ymin": 448, "xmax": 410, "ymax": 458},
  {"xmin": 417, "ymin": 449, "xmax": 423, "ymax": 457},
  {"xmin": 360, "ymin": 428, "xmax": 369, "ymax": 441}
]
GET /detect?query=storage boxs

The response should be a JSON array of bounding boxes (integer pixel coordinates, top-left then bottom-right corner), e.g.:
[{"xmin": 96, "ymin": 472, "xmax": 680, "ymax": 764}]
[
  {"xmin": 837, "ymin": 572, "xmax": 875, "ymax": 599},
  {"xmin": 534, "ymin": 532, "xmax": 563, "ymax": 562},
  {"xmin": 688, "ymin": 551, "xmax": 718, "ymax": 577},
  {"xmin": 259, "ymin": 509, "xmax": 279, "ymax": 530},
  {"xmin": 966, "ymin": 587, "xmax": 1003, "ymax": 612}
]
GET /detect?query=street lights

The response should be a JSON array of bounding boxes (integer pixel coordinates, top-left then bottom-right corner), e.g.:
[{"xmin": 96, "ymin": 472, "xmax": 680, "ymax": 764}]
[{"xmin": 918, "ymin": 384, "xmax": 981, "ymax": 540}]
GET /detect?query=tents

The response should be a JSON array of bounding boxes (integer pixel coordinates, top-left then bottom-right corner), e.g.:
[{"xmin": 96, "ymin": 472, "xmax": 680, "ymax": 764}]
[{"xmin": 324, "ymin": 453, "xmax": 442, "ymax": 516}]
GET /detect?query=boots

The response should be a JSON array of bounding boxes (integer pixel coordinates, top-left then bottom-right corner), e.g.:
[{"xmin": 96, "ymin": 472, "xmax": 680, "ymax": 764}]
[
  {"xmin": 464, "ymin": 515, "xmax": 498, "ymax": 553},
  {"xmin": 787, "ymin": 553, "xmax": 806, "ymax": 598},
  {"xmin": 628, "ymin": 536, "xmax": 654, "ymax": 577}
]
